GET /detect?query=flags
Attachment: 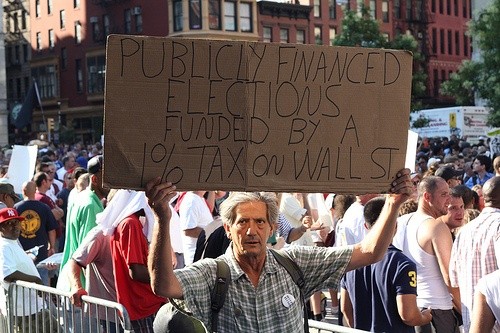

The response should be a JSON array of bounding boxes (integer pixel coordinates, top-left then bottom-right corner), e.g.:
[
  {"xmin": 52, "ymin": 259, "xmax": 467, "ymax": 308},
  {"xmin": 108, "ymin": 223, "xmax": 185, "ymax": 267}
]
[{"xmin": 14, "ymin": 83, "xmax": 38, "ymax": 133}]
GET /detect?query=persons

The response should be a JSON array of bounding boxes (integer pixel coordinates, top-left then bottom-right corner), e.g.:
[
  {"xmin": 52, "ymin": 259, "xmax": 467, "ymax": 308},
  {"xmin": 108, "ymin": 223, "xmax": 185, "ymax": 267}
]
[
  {"xmin": 145, "ymin": 168, "xmax": 413, "ymax": 333},
  {"xmin": 0, "ymin": 139, "xmax": 230, "ymax": 333},
  {"xmin": 256, "ymin": 135, "xmax": 500, "ymax": 333}
]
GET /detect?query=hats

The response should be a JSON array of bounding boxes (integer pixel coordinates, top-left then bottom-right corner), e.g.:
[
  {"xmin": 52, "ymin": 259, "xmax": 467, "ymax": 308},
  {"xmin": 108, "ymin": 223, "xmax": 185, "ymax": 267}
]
[
  {"xmin": 0, "ymin": 184, "xmax": 21, "ymax": 201},
  {"xmin": 76, "ymin": 157, "xmax": 87, "ymax": 168},
  {"xmin": 417, "ymin": 151, "xmax": 428, "ymax": 160},
  {"xmin": 0, "ymin": 208, "xmax": 25, "ymax": 225},
  {"xmin": 478, "ymin": 146, "xmax": 486, "ymax": 152},
  {"xmin": 87, "ymin": 154, "xmax": 102, "ymax": 175},
  {"xmin": 72, "ymin": 167, "xmax": 86, "ymax": 178},
  {"xmin": 479, "ymin": 137, "xmax": 483, "ymax": 140},
  {"xmin": 427, "ymin": 158, "xmax": 440, "ymax": 169},
  {"xmin": 434, "ymin": 163, "xmax": 464, "ymax": 182},
  {"xmin": 444, "ymin": 148, "xmax": 450, "ymax": 154}
]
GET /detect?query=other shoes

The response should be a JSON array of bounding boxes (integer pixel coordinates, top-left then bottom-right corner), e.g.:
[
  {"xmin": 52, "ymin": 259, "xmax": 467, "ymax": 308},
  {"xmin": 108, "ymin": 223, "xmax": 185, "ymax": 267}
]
[{"xmin": 331, "ymin": 306, "xmax": 338, "ymax": 315}]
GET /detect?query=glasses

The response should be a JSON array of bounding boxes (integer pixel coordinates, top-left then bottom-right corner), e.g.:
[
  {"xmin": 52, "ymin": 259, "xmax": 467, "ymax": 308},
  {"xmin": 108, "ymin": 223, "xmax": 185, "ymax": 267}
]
[{"xmin": 44, "ymin": 170, "xmax": 50, "ymax": 174}]
